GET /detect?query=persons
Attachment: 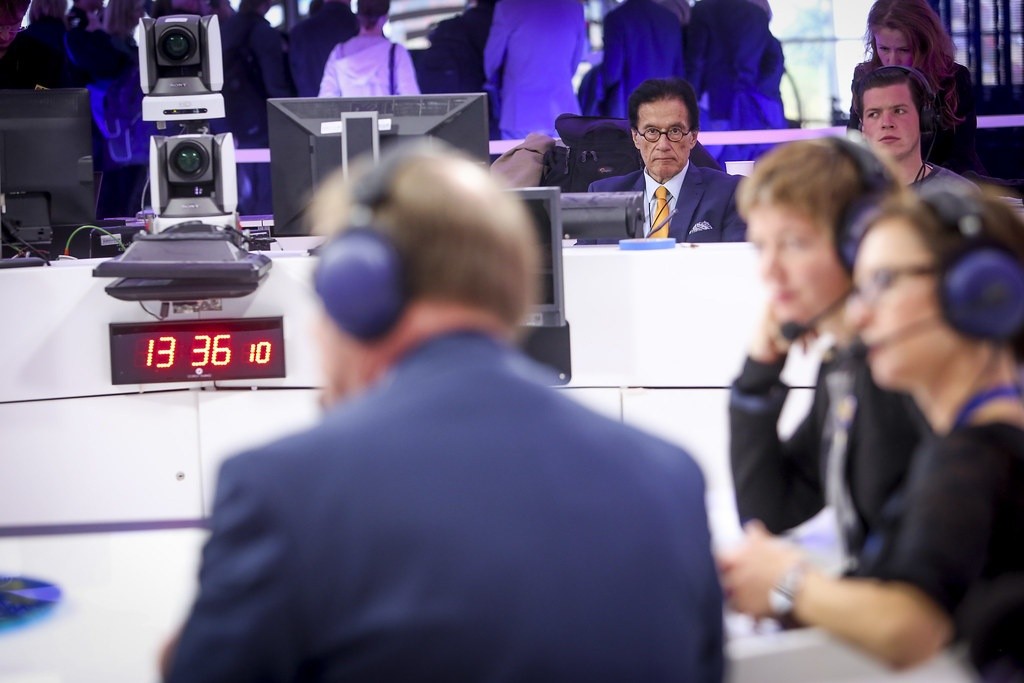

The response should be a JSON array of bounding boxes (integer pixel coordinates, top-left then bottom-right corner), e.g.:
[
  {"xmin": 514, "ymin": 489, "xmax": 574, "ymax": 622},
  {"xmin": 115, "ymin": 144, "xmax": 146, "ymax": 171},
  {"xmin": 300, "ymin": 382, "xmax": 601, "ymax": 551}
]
[
  {"xmin": 165, "ymin": 135, "xmax": 725, "ymax": 682},
  {"xmin": 573, "ymin": 79, "xmax": 748, "ymax": 245},
  {"xmin": 848, "ymin": 0, "xmax": 983, "ymax": 172},
  {"xmin": 853, "ymin": 68, "xmax": 981, "ymax": 194},
  {"xmin": 729, "ymin": 138, "xmax": 922, "ymax": 557},
  {"xmin": 713, "ymin": 189, "xmax": 1024, "ymax": 683},
  {"xmin": 0, "ymin": 0, "xmax": 786, "ymax": 260}
]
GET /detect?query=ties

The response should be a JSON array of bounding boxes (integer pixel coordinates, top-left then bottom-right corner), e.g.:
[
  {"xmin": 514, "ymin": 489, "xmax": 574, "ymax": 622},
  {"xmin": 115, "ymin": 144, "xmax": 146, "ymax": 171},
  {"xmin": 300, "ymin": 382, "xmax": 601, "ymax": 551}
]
[
  {"xmin": 824, "ymin": 373, "xmax": 858, "ymax": 531},
  {"xmin": 650, "ymin": 187, "xmax": 670, "ymax": 239}
]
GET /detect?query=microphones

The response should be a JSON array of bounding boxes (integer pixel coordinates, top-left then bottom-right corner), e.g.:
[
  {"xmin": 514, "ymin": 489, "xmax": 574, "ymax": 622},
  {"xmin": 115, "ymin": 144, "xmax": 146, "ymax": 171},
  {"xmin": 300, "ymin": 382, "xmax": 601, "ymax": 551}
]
[
  {"xmin": 848, "ymin": 314, "xmax": 942, "ymax": 359},
  {"xmin": 646, "ymin": 209, "xmax": 678, "ymax": 238},
  {"xmin": 780, "ymin": 288, "xmax": 854, "ymax": 340}
]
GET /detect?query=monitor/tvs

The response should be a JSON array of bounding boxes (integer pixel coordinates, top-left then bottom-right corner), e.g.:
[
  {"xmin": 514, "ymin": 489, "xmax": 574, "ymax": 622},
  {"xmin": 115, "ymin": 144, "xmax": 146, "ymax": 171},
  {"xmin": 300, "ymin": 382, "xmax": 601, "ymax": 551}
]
[
  {"xmin": 0, "ymin": 88, "xmax": 95, "ymax": 243},
  {"xmin": 512, "ymin": 186, "xmax": 566, "ymax": 326},
  {"xmin": 267, "ymin": 92, "xmax": 489, "ymax": 235}
]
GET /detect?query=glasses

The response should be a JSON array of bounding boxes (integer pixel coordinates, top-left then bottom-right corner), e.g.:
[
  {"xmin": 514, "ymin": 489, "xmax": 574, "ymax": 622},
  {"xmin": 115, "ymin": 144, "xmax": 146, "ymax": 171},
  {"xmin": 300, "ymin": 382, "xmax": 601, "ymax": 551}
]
[
  {"xmin": 873, "ymin": 265, "xmax": 937, "ymax": 297},
  {"xmin": 636, "ymin": 127, "xmax": 692, "ymax": 142}
]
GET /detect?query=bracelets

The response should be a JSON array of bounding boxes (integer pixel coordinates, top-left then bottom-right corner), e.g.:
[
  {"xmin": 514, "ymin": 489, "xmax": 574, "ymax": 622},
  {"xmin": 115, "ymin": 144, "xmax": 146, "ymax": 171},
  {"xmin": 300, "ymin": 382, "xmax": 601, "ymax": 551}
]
[{"xmin": 770, "ymin": 575, "xmax": 803, "ymax": 630}]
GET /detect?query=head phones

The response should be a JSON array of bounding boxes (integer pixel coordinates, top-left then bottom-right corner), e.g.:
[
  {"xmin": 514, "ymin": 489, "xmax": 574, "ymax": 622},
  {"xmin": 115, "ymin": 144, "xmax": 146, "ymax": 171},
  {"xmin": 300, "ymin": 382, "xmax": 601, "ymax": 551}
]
[
  {"xmin": 878, "ymin": 65, "xmax": 937, "ymax": 132},
  {"xmin": 831, "ymin": 137, "xmax": 891, "ymax": 272},
  {"xmin": 312, "ymin": 137, "xmax": 417, "ymax": 340},
  {"xmin": 923, "ymin": 190, "xmax": 1023, "ymax": 337}
]
